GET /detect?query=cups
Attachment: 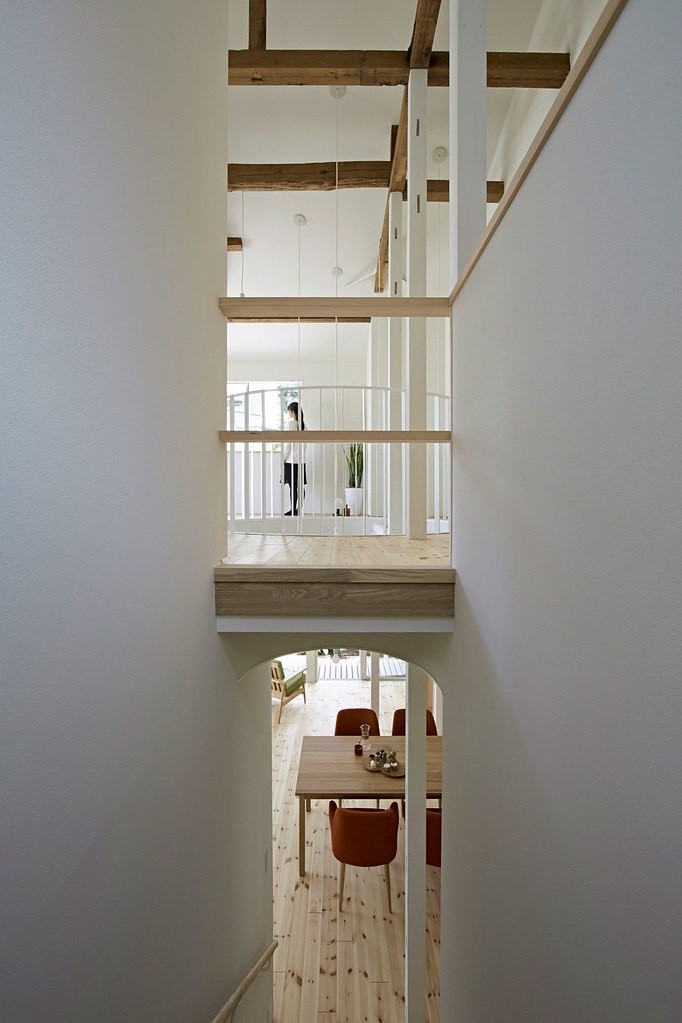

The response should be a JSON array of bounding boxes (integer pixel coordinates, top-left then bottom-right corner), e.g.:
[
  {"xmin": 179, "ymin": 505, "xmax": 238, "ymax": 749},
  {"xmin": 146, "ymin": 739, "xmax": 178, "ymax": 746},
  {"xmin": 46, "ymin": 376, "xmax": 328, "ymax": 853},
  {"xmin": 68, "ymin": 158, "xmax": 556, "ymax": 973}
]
[{"xmin": 354, "ymin": 744, "xmax": 363, "ymax": 756}]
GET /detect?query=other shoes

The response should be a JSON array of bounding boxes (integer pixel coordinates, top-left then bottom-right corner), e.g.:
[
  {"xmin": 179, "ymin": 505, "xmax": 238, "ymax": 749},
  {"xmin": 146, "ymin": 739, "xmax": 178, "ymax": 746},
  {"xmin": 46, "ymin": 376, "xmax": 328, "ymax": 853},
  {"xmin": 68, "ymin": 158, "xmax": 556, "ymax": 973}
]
[{"xmin": 284, "ymin": 510, "xmax": 298, "ymax": 516}]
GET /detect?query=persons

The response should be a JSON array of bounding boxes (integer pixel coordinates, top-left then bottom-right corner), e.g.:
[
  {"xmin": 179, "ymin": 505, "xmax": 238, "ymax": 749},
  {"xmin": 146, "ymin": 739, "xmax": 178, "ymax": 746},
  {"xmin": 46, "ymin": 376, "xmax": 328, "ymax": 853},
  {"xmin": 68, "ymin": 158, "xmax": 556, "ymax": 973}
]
[{"xmin": 283, "ymin": 402, "xmax": 307, "ymax": 515}]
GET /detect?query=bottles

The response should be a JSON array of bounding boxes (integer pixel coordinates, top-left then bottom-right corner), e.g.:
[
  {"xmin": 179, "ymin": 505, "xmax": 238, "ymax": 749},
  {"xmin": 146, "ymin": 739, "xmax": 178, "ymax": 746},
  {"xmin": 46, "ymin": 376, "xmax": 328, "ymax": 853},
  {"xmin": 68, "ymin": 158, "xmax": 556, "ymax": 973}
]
[
  {"xmin": 343, "ymin": 504, "xmax": 351, "ymax": 516},
  {"xmin": 368, "ymin": 749, "xmax": 388, "ymax": 769}
]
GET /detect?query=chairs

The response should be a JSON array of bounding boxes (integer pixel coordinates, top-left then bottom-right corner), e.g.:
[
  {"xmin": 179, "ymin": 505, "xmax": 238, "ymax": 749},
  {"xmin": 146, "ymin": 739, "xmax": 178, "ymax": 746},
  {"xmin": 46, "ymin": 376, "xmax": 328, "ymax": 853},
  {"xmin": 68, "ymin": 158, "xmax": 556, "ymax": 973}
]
[
  {"xmin": 334, "ymin": 708, "xmax": 381, "ymax": 810},
  {"xmin": 271, "ymin": 660, "xmax": 308, "ymax": 724},
  {"xmin": 403, "ymin": 801, "xmax": 442, "ymax": 868},
  {"xmin": 329, "ymin": 800, "xmax": 399, "ymax": 914},
  {"xmin": 392, "ymin": 708, "xmax": 442, "ymax": 817}
]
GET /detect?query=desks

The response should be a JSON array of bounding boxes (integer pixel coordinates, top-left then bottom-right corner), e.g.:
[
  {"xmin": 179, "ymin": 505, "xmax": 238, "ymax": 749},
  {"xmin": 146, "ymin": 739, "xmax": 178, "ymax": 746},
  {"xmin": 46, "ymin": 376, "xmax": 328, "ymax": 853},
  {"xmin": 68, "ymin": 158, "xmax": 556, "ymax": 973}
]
[{"xmin": 296, "ymin": 734, "xmax": 442, "ymax": 876}]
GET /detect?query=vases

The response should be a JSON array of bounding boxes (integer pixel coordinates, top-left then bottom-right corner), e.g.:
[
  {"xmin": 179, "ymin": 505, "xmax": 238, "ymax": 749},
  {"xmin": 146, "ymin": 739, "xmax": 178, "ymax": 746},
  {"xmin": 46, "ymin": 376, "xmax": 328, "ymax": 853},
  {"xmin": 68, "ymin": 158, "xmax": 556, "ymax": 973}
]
[{"xmin": 359, "ymin": 724, "xmax": 371, "ymax": 751}]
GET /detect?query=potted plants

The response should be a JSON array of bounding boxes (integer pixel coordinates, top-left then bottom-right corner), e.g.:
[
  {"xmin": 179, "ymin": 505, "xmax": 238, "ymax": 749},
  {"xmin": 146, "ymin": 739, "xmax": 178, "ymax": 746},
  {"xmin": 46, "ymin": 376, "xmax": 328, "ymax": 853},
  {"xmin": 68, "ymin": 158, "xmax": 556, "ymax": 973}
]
[{"xmin": 343, "ymin": 443, "xmax": 365, "ymax": 516}]
